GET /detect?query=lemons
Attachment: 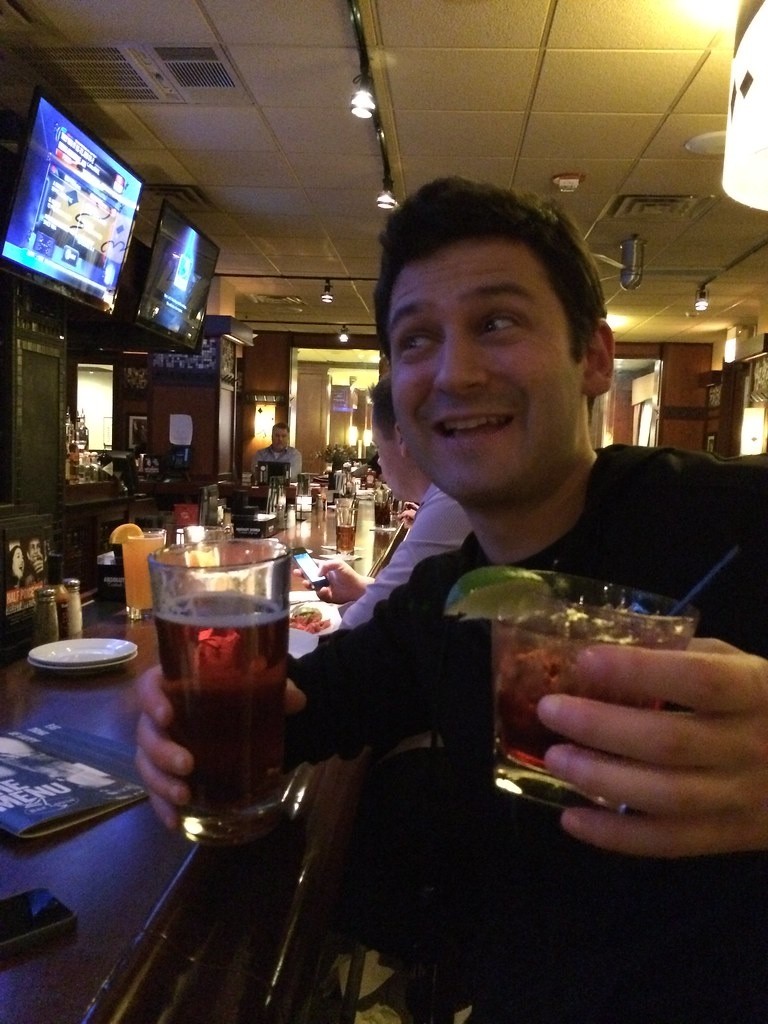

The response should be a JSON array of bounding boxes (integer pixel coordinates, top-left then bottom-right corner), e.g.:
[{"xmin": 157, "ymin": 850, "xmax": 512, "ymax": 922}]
[
  {"xmin": 109, "ymin": 524, "xmax": 145, "ymax": 544},
  {"xmin": 442, "ymin": 564, "xmax": 559, "ymax": 620}
]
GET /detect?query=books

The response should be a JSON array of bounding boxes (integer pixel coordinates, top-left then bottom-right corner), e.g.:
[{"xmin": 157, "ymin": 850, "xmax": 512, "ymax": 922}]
[{"xmin": 0, "ymin": 722, "xmax": 146, "ymax": 837}]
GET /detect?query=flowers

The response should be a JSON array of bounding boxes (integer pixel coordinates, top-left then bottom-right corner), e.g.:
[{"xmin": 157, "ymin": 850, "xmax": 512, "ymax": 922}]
[{"xmin": 323, "ymin": 443, "xmax": 357, "ymax": 464}]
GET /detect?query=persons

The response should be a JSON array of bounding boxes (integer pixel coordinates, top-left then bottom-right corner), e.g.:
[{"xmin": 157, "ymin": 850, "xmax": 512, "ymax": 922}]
[
  {"xmin": 250, "ymin": 423, "xmax": 302, "ymax": 482},
  {"xmin": 397, "ymin": 501, "xmax": 419, "ymax": 520},
  {"xmin": 134, "ymin": 177, "xmax": 768, "ymax": 1023},
  {"xmin": 9, "ymin": 538, "xmax": 43, "ymax": 588},
  {"xmin": 293, "ymin": 370, "xmax": 472, "ymax": 629}
]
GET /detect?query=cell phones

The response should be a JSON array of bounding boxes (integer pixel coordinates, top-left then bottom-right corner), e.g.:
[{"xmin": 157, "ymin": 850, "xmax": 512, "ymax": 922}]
[
  {"xmin": 0, "ymin": 887, "xmax": 78, "ymax": 961},
  {"xmin": 289, "ymin": 547, "xmax": 328, "ymax": 586}
]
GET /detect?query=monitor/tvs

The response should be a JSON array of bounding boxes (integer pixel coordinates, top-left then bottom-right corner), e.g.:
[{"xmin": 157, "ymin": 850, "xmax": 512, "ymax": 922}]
[
  {"xmin": 2, "ymin": 85, "xmax": 147, "ymax": 315},
  {"xmin": 133, "ymin": 198, "xmax": 220, "ymax": 355}
]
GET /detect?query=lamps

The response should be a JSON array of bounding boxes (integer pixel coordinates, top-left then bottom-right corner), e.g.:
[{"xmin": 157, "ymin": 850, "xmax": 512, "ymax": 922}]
[
  {"xmin": 694, "ymin": 290, "xmax": 709, "ymax": 310},
  {"xmin": 322, "ymin": 279, "xmax": 333, "ymax": 304},
  {"xmin": 351, "ymin": 64, "xmax": 376, "ymax": 119},
  {"xmin": 340, "ymin": 325, "xmax": 349, "ymax": 342},
  {"xmin": 376, "ymin": 177, "xmax": 397, "ymax": 211},
  {"xmin": 559, "ymin": 178, "xmax": 579, "ymax": 192}
]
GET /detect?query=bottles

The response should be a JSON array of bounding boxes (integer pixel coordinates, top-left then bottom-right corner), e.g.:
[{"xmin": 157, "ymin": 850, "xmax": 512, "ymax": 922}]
[
  {"xmin": 275, "ymin": 505, "xmax": 286, "ymax": 531},
  {"xmin": 32, "ymin": 588, "xmax": 58, "ymax": 646},
  {"xmin": 223, "ymin": 508, "xmax": 231, "ymax": 526},
  {"xmin": 67, "ymin": 411, "xmax": 101, "ymax": 486},
  {"xmin": 59, "ymin": 579, "xmax": 82, "ymax": 642},
  {"xmin": 316, "ymin": 494, "xmax": 324, "ymax": 513},
  {"xmin": 286, "ymin": 504, "xmax": 296, "ymax": 530}
]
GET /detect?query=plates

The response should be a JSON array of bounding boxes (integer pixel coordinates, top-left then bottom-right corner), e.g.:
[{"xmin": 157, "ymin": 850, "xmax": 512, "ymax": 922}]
[
  {"xmin": 289, "ymin": 591, "xmax": 341, "ymax": 659},
  {"xmin": 27, "ymin": 639, "xmax": 139, "ymax": 670}
]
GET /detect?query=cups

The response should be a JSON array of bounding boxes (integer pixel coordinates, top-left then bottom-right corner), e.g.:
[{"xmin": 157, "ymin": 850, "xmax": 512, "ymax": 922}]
[
  {"xmin": 122, "ymin": 527, "xmax": 168, "ymax": 621},
  {"xmin": 148, "ymin": 540, "xmax": 293, "ymax": 849},
  {"xmin": 335, "ymin": 463, "xmax": 390, "ymax": 553},
  {"xmin": 176, "ymin": 525, "xmax": 233, "ymax": 547},
  {"xmin": 489, "ymin": 571, "xmax": 699, "ymax": 809}
]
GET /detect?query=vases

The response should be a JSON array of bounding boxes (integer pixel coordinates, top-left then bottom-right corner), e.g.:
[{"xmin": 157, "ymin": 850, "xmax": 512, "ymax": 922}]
[{"xmin": 329, "ymin": 462, "xmax": 343, "ymax": 489}]
